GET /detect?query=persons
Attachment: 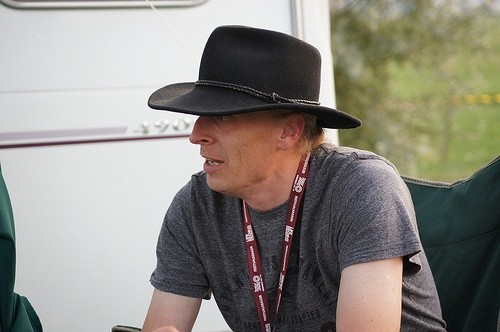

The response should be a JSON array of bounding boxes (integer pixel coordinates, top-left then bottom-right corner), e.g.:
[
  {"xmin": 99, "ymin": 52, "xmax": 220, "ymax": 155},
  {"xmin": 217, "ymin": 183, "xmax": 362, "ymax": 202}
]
[{"xmin": 140, "ymin": 24, "xmax": 447, "ymax": 332}]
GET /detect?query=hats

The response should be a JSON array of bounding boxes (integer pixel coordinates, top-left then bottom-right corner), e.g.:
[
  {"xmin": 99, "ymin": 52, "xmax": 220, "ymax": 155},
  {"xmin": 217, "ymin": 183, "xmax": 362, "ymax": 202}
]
[{"xmin": 148, "ymin": 25, "xmax": 362, "ymax": 129}]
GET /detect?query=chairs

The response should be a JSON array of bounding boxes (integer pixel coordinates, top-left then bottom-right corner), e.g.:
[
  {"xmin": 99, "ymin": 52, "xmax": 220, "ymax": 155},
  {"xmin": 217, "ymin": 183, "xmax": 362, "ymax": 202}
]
[{"xmin": 394, "ymin": 152, "xmax": 500, "ymax": 332}]
